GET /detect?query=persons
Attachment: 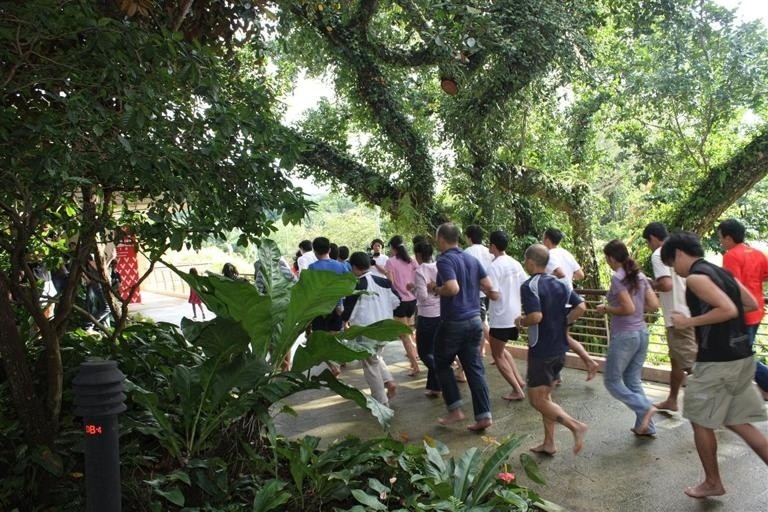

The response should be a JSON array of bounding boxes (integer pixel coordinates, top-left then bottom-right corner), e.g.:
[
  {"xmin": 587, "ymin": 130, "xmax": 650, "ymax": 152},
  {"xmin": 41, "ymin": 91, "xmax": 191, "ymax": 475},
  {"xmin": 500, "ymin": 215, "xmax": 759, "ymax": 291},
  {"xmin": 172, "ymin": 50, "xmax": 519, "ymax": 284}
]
[
  {"xmin": 19, "ymin": 225, "xmax": 123, "ymax": 335},
  {"xmin": 292, "ymin": 223, "xmax": 657, "ymax": 455},
  {"xmin": 221, "ymin": 262, "xmax": 239, "ymax": 281},
  {"xmin": 187, "ymin": 267, "xmax": 207, "ymax": 320},
  {"xmin": 255, "ymin": 254, "xmax": 299, "ymax": 373},
  {"xmin": 660, "ymin": 228, "xmax": 768, "ymax": 498},
  {"xmin": 712, "ymin": 218, "xmax": 767, "ymax": 396},
  {"xmin": 640, "ymin": 222, "xmax": 698, "ymax": 412}
]
